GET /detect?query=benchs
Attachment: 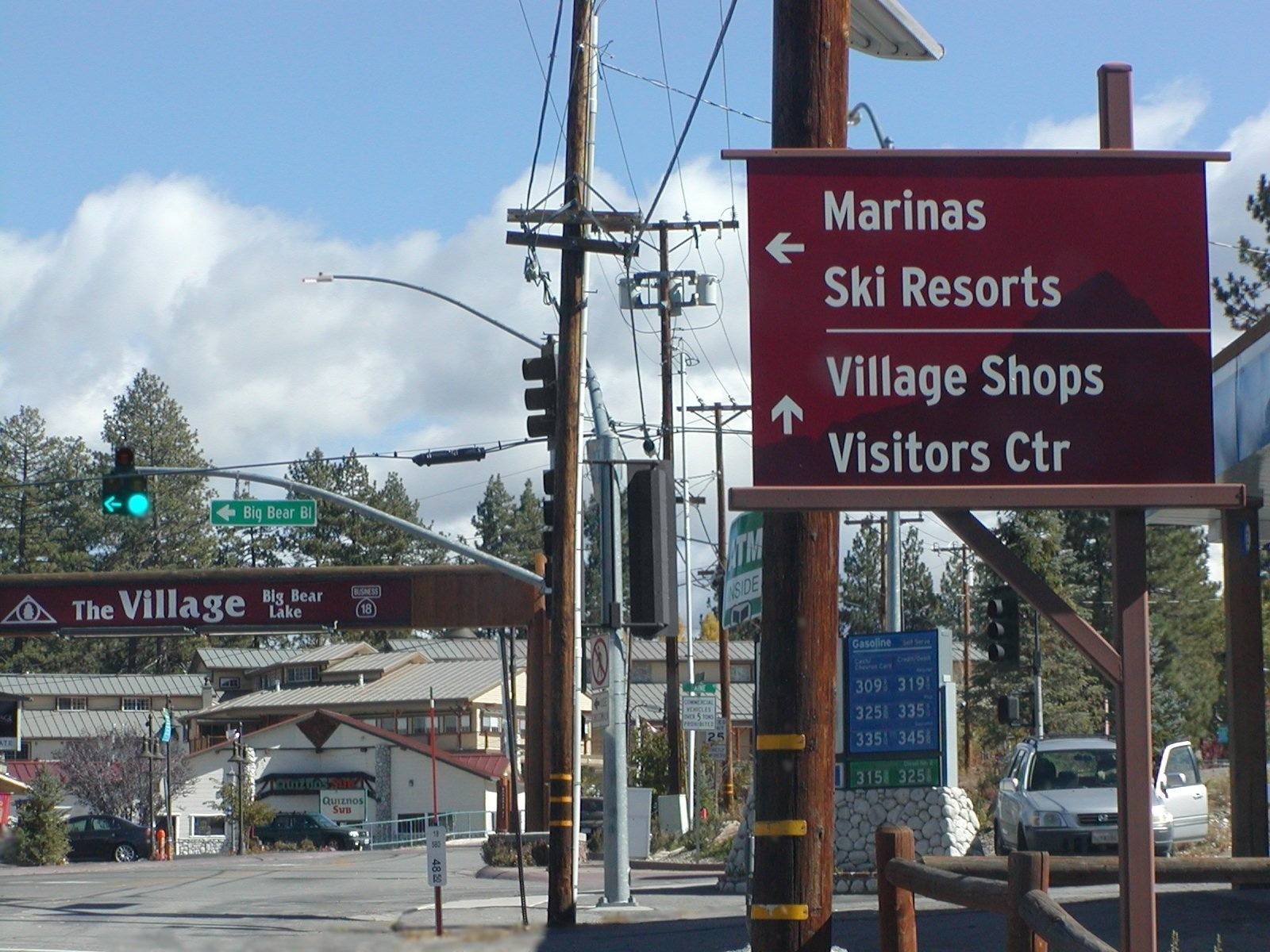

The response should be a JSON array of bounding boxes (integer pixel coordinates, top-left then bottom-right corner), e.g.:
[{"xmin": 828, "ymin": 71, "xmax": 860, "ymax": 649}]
[{"xmin": 1056, "ymin": 770, "xmax": 1098, "ymax": 788}]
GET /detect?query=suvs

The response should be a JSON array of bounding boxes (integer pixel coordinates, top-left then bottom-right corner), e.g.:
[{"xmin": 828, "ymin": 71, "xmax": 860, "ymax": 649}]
[{"xmin": 998, "ymin": 734, "xmax": 1209, "ymax": 859}]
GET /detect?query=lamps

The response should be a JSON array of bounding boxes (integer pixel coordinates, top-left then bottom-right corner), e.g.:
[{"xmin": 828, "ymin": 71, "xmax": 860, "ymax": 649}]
[{"xmin": 408, "ymin": 780, "xmax": 413, "ymax": 786}]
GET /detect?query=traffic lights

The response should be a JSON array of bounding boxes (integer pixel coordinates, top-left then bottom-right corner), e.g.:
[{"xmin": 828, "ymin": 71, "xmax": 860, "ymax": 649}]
[
  {"xmin": 98, "ymin": 448, "xmax": 152, "ymax": 518},
  {"xmin": 209, "ymin": 500, "xmax": 316, "ymax": 527},
  {"xmin": 987, "ymin": 586, "xmax": 1019, "ymax": 672},
  {"xmin": 522, "ymin": 338, "xmax": 559, "ymax": 452},
  {"xmin": 538, "ymin": 465, "xmax": 557, "ymax": 621},
  {"xmin": 998, "ymin": 695, "xmax": 1018, "ymax": 723}
]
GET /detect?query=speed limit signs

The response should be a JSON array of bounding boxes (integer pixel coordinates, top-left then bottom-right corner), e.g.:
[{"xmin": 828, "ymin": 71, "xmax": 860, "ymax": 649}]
[{"xmin": 706, "ymin": 720, "xmax": 727, "ymax": 745}]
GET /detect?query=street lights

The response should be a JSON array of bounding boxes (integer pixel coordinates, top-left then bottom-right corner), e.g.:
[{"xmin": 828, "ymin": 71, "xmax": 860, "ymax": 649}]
[
  {"xmin": 139, "ymin": 713, "xmax": 166, "ymax": 859},
  {"xmin": 302, "ymin": 271, "xmax": 631, "ymax": 907},
  {"xmin": 229, "ymin": 720, "xmax": 253, "ymax": 856}
]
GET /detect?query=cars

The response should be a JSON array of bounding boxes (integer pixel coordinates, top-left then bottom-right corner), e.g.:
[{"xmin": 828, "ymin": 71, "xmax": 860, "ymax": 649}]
[
  {"xmin": 251, "ymin": 811, "xmax": 370, "ymax": 851},
  {"xmin": 59, "ymin": 813, "xmax": 157, "ymax": 863},
  {"xmin": 580, "ymin": 795, "xmax": 606, "ymax": 838}
]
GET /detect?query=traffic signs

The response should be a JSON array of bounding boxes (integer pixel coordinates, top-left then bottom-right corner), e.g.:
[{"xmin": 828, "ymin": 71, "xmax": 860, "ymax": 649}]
[{"xmin": 716, "ymin": 147, "xmax": 1242, "ymax": 516}]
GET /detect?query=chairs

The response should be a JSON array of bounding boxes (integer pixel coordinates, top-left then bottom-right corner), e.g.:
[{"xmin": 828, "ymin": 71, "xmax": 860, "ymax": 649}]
[
  {"xmin": 1096, "ymin": 757, "xmax": 1118, "ymax": 783},
  {"xmin": 1036, "ymin": 758, "xmax": 1057, "ymax": 780}
]
